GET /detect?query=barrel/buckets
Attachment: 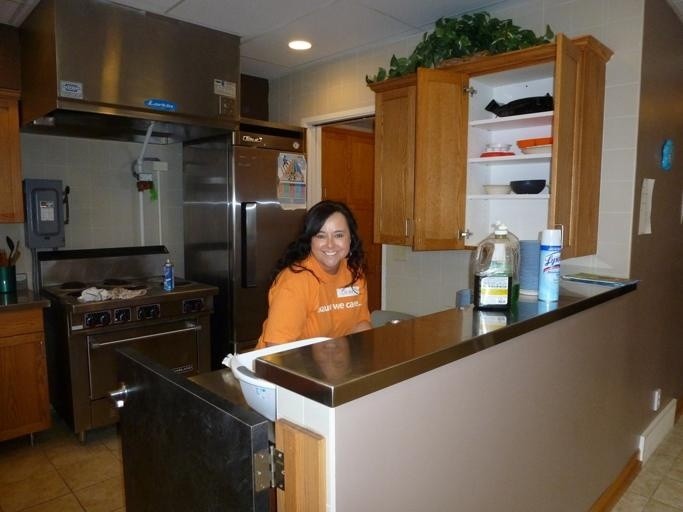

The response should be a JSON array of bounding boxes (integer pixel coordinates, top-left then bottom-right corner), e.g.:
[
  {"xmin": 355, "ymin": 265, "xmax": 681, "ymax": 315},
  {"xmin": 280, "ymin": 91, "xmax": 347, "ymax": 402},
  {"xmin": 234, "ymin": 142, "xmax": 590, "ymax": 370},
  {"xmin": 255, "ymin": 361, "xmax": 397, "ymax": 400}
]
[
  {"xmin": 470, "ymin": 229, "xmax": 513, "ymax": 311},
  {"xmin": 483, "ymin": 223, "xmax": 522, "ymax": 304}
]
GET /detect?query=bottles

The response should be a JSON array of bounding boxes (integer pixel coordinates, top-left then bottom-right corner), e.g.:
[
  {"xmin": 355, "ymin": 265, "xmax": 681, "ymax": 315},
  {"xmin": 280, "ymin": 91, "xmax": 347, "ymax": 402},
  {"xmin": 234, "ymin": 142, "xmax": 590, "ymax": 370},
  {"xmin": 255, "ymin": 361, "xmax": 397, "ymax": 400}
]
[{"xmin": 535, "ymin": 226, "xmax": 563, "ymax": 306}]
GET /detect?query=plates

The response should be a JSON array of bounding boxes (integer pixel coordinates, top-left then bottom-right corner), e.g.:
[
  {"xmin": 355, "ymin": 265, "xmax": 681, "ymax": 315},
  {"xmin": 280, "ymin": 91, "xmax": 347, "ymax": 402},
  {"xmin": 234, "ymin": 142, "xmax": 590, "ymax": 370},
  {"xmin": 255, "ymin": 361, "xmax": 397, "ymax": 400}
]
[
  {"xmin": 480, "ymin": 152, "xmax": 515, "ymax": 157},
  {"xmin": 516, "ymin": 137, "xmax": 553, "ymax": 154}
]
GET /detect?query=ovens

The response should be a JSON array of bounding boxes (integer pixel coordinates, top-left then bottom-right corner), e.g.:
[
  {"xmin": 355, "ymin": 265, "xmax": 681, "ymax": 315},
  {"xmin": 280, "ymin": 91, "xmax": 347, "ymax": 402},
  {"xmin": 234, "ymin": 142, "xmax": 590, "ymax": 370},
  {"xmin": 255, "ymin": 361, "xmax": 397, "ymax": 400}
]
[{"xmin": 67, "ymin": 295, "xmax": 215, "ymax": 444}]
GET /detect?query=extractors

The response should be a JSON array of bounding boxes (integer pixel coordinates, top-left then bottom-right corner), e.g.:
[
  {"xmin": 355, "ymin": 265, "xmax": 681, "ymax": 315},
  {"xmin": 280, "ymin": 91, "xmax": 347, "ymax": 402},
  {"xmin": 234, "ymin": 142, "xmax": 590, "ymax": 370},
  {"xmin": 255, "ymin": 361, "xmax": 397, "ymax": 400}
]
[{"xmin": 17, "ymin": 0, "xmax": 246, "ymax": 146}]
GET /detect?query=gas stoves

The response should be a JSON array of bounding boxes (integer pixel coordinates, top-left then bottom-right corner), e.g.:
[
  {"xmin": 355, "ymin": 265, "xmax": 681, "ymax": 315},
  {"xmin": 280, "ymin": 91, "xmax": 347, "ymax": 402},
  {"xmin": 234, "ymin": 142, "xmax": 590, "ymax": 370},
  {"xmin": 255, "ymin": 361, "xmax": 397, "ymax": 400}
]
[{"xmin": 43, "ymin": 274, "xmax": 221, "ymax": 314}]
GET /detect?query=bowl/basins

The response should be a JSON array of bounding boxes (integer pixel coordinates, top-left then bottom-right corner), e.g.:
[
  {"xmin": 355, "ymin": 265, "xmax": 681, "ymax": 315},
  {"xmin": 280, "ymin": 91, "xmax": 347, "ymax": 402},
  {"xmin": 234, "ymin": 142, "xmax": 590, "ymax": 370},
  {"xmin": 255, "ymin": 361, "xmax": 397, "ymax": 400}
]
[
  {"xmin": 482, "ymin": 185, "xmax": 511, "ymax": 194},
  {"xmin": 510, "ymin": 180, "xmax": 546, "ymax": 194},
  {"xmin": 486, "ymin": 144, "xmax": 511, "ymax": 152}
]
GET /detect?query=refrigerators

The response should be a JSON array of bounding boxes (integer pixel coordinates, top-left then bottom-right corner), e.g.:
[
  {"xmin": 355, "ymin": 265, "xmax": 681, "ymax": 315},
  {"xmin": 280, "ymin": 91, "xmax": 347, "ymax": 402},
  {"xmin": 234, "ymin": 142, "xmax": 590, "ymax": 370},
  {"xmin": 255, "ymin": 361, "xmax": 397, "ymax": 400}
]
[{"xmin": 181, "ymin": 129, "xmax": 307, "ymax": 371}]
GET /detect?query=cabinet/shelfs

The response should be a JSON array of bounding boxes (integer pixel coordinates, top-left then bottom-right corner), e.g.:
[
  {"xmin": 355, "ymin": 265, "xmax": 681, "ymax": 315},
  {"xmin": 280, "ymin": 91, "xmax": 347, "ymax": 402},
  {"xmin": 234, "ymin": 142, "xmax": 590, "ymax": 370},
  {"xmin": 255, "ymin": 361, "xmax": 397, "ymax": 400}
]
[
  {"xmin": 368, "ymin": 35, "xmax": 613, "ymax": 260},
  {"xmin": 0, "ymin": 309, "xmax": 52, "ymax": 446}
]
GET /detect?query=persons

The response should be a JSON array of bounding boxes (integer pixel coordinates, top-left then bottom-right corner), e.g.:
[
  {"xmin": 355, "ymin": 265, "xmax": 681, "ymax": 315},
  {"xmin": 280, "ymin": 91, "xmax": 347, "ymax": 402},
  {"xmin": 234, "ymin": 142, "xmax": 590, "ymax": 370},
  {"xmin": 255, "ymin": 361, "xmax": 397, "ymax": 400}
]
[{"xmin": 252, "ymin": 200, "xmax": 371, "ymax": 350}]
